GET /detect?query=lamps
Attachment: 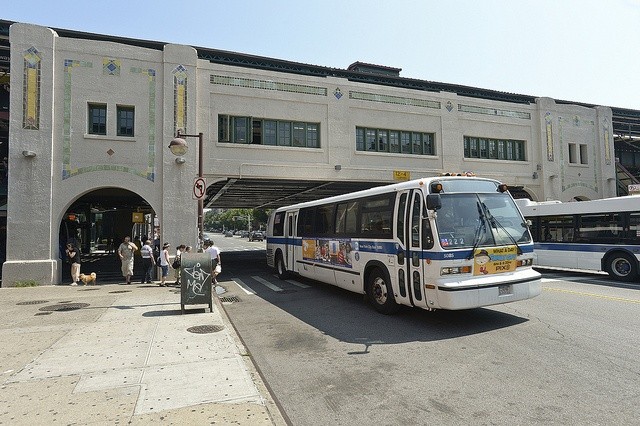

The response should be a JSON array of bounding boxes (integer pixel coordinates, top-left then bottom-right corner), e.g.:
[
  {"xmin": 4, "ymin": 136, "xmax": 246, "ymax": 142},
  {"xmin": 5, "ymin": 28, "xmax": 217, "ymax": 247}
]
[
  {"xmin": 23, "ymin": 150, "xmax": 36, "ymax": 158},
  {"xmin": 175, "ymin": 158, "xmax": 185, "ymax": 163}
]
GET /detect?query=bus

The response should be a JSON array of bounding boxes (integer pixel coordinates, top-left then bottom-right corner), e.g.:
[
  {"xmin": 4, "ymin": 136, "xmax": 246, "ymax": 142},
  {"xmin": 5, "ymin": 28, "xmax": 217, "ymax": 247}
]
[
  {"xmin": 514, "ymin": 192, "xmax": 640, "ymax": 284},
  {"xmin": 265, "ymin": 171, "xmax": 543, "ymax": 315}
]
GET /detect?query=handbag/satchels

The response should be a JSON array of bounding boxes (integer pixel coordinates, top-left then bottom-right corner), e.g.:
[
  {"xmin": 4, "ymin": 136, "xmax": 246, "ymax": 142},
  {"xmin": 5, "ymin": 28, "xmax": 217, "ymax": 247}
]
[
  {"xmin": 172, "ymin": 260, "xmax": 181, "ymax": 268},
  {"xmin": 156, "ymin": 257, "xmax": 161, "ymax": 266}
]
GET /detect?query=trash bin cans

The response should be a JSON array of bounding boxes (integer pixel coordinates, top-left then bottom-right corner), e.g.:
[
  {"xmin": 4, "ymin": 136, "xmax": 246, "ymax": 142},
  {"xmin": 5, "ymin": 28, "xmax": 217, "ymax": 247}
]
[{"xmin": 180, "ymin": 252, "xmax": 213, "ymax": 312}]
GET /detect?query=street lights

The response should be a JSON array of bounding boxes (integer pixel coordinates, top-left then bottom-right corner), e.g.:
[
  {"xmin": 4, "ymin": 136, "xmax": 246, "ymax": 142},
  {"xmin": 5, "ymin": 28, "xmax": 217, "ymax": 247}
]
[{"xmin": 166, "ymin": 128, "xmax": 204, "ymax": 252}]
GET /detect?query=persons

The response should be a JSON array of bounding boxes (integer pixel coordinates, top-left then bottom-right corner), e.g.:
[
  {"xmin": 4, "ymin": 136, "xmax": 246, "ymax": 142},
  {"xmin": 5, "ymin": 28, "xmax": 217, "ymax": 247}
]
[
  {"xmin": 66, "ymin": 243, "xmax": 81, "ymax": 285},
  {"xmin": 140, "ymin": 240, "xmax": 156, "ymax": 284},
  {"xmin": 118, "ymin": 237, "xmax": 138, "ymax": 284},
  {"xmin": 141, "ymin": 235, "xmax": 148, "ymax": 246},
  {"xmin": 185, "ymin": 246, "xmax": 192, "ymax": 253},
  {"xmin": 160, "ymin": 242, "xmax": 172, "ymax": 287},
  {"xmin": 132, "ymin": 236, "xmax": 142, "ymax": 256},
  {"xmin": 205, "ymin": 240, "xmax": 221, "ymax": 284},
  {"xmin": 175, "ymin": 243, "xmax": 185, "ymax": 284}
]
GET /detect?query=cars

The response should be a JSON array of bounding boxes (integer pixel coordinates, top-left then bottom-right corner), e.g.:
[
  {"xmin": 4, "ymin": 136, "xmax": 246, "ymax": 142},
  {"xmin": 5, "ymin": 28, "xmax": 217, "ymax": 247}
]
[
  {"xmin": 249, "ymin": 231, "xmax": 264, "ymax": 242},
  {"xmin": 241, "ymin": 231, "xmax": 250, "ymax": 238}
]
